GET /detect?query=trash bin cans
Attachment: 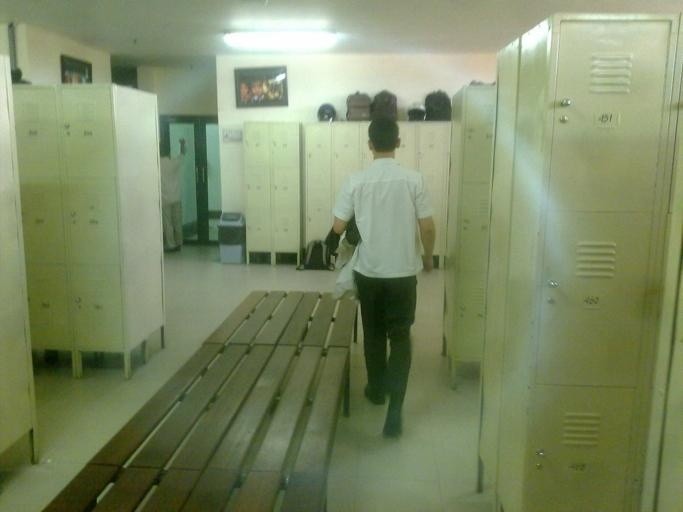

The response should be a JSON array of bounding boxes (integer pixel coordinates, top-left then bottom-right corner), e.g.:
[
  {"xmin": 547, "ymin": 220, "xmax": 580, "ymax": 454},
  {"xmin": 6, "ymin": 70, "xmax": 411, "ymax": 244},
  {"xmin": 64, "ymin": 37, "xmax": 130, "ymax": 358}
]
[{"xmin": 217, "ymin": 212, "xmax": 246, "ymax": 264}]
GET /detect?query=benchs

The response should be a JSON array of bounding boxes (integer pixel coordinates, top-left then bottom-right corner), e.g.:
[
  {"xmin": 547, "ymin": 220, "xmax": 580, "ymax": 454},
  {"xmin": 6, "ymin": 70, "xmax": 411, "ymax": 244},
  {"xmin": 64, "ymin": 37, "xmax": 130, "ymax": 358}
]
[{"xmin": 35, "ymin": 293, "xmax": 357, "ymax": 512}]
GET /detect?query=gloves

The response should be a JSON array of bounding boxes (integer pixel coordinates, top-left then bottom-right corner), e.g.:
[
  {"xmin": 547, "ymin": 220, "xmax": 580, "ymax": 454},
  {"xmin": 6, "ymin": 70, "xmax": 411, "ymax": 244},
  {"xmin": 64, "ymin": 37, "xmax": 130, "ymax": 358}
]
[{"xmin": 325, "ymin": 227, "xmax": 340, "ymax": 251}]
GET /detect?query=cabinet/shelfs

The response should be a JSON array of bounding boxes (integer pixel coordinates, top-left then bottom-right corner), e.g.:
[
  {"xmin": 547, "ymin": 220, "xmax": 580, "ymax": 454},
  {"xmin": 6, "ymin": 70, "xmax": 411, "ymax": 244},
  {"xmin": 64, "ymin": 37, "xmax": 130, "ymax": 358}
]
[
  {"xmin": 12, "ymin": 57, "xmax": 164, "ymax": 381},
  {"xmin": 239, "ymin": 118, "xmax": 450, "ymax": 268},
  {"xmin": 443, "ymin": 11, "xmax": 677, "ymax": 507},
  {"xmin": 1, "ymin": 57, "xmax": 51, "ymax": 466}
]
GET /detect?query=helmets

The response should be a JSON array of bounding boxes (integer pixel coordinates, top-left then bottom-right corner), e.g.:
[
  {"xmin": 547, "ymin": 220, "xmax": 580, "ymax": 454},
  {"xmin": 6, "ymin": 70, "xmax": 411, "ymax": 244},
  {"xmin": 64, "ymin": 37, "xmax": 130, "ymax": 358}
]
[{"xmin": 317, "ymin": 103, "xmax": 336, "ymax": 121}]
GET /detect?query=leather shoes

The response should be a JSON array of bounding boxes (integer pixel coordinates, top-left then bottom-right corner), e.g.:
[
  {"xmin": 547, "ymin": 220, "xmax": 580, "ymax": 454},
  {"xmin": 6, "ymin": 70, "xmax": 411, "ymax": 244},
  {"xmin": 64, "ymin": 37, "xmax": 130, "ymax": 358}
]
[
  {"xmin": 383, "ymin": 420, "xmax": 403, "ymax": 438},
  {"xmin": 364, "ymin": 385, "xmax": 385, "ymax": 404}
]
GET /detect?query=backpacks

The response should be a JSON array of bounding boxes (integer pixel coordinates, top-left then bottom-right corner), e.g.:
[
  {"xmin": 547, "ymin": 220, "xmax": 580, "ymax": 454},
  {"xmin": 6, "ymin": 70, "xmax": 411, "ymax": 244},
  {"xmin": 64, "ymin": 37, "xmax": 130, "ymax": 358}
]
[
  {"xmin": 296, "ymin": 239, "xmax": 339, "ymax": 272},
  {"xmin": 407, "ymin": 89, "xmax": 452, "ymax": 121},
  {"xmin": 345, "ymin": 89, "xmax": 398, "ymax": 121}
]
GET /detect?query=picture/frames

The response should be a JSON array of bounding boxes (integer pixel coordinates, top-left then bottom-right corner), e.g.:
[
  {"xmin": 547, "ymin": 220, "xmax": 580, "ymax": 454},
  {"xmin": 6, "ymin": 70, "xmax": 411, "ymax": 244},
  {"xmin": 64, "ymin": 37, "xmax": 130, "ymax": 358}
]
[{"xmin": 233, "ymin": 66, "xmax": 288, "ymax": 107}]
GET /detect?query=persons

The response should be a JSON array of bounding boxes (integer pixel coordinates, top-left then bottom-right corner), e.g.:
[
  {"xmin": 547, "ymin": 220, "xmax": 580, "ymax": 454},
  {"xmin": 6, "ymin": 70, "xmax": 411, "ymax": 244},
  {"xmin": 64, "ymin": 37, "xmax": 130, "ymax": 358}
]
[
  {"xmin": 333, "ymin": 117, "xmax": 435, "ymax": 440},
  {"xmin": 160, "ymin": 139, "xmax": 186, "ymax": 252}
]
